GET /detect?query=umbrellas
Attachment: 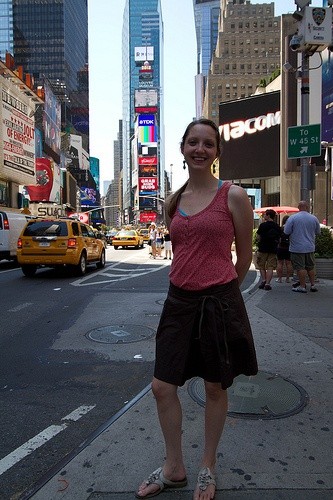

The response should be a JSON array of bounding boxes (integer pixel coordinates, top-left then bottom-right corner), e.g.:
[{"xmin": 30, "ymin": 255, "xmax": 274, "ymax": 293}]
[{"xmin": 253, "ymin": 204, "xmax": 300, "ymax": 225}]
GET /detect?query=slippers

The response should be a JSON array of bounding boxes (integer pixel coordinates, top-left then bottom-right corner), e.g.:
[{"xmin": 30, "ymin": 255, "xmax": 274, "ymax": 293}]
[
  {"xmin": 193, "ymin": 470, "xmax": 217, "ymax": 500},
  {"xmin": 134, "ymin": 467, "xmax": 188, "ymax": 499}
]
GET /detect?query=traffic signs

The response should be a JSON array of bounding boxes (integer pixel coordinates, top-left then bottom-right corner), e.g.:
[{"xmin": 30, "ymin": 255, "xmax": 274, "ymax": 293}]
[{"xmin": 286, "ymin": 123, "xmax": 322, "ymax": 158}]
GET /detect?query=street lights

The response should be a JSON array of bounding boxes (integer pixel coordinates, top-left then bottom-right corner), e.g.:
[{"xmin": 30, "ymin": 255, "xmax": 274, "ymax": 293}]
[{"xmin": 59, "ymin": 168, "xmax": 92, "ymax": 201}]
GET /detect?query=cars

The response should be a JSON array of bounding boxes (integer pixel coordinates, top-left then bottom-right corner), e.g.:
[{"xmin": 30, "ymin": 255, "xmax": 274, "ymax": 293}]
[
  {"xmin": 138, "ymin": 228, "xmax": 150, "ymax": 241},
  {"xmin": 16, "ymin": 219, "xmax": 106, "ymax": 278},
  {"xmin": 105, "ymin": 231, "xmax": 118, "ymax": 246},
  {"xmin": 112, "ymin": 229, "xmax": 144, "ymax": 249}
]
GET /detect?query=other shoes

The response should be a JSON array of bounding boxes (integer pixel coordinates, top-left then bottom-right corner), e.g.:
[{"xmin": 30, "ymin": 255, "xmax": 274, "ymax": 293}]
[
  {"xmin": 292, "ymin": 286, "xmax": 307, "ymax": 293},
  {"xmin": 264, "ymin": 285, "xmax": 272, "ymax": 290},
  {"xmin": 310, "ymin": 287, "xmax": 318, "ymax": 292},
  {"xmin": 293, "ymin": 281, "xmax": 306, "ymax": 288},
  {"xmin": 259, "ymin": 281, "xmax": 265, "ymax": 290},
  {"xmin": 276, "ymin": 279, "xmax": 282, "ymax": 283},
  {"xmin": 285, "ymin": 278, "xmax": 290, "ymax": 283}
]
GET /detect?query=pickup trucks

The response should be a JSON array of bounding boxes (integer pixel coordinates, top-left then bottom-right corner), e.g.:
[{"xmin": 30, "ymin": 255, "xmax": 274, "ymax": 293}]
[{"xmin": 0, "ymin": 211, "xmax": 36, "ymax": 267}]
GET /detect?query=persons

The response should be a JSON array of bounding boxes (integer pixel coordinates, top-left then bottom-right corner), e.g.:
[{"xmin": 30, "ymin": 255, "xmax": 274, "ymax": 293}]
[
  {"xmin": 256, "ymin": 208, "xmax": 281, "ymax": 291},
  {"xmin": 133, "ymin": 117, "xmax": 259, "ymax": 500},
  {"xmin": 97, "ymin": 231, "xmax": 102, "ymax": 239},
  {"xmin": 276, "ymin": 215, "xmax": 292, "ymax": 283},
  {"xmin": 283, "ymin": 200, "xmax": 321, "ymax": 293},
  {"xmin": 146, "ymin": 222, "xmax": 173, "ymax": 260}
]
